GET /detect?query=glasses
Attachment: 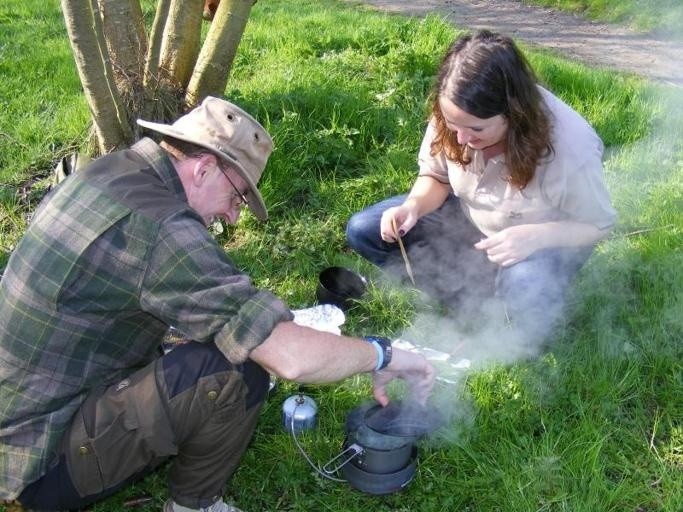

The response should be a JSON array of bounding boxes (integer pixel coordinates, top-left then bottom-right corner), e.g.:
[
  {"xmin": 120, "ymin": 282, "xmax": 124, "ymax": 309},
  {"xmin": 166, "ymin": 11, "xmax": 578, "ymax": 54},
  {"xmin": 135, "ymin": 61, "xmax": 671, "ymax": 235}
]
[{"xmin": 218, "ymin": 164, "xmax": 251, "ymax": 210}]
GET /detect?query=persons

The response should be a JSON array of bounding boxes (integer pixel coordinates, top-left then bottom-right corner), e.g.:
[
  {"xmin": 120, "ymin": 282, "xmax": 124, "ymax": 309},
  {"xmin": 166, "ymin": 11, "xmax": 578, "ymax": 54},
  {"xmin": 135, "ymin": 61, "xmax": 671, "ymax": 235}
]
[
  {"xmin": 341, "ymin": 25, "xmax": 619, "ymax": 366},
  {"xmin": 0, "ymin": 93, "xmax": 440, "ymax": 512}
]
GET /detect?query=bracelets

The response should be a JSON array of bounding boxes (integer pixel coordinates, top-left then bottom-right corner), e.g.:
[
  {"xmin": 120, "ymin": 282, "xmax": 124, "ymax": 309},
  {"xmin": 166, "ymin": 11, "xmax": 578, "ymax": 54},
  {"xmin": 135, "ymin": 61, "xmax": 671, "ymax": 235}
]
[{"xmin": 362, "ymin": 333, "xmax": 394, "ymax": 373}]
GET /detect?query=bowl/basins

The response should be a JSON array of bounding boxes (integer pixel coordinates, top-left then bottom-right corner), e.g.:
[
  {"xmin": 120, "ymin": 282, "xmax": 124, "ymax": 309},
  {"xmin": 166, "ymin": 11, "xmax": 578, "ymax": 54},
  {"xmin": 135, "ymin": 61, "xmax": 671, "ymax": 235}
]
[{"xmin": 316, "ymin": 266, "xmax": 367, "ymax": 311}]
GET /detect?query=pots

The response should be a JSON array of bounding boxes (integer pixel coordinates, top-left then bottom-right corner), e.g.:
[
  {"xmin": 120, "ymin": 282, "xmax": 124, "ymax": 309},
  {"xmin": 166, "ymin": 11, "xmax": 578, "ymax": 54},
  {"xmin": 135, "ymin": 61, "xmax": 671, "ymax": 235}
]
[{"xmin": 323, "ymin": 404, "xmax": 415, "ymax": 474}]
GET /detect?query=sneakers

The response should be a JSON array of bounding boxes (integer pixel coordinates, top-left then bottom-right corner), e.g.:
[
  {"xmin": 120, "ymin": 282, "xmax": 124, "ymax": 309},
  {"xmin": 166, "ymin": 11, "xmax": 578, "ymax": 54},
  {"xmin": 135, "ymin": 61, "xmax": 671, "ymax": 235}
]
[{"xmin": 163, "ymin": 496, "xmax": 243, "ymax": 512}]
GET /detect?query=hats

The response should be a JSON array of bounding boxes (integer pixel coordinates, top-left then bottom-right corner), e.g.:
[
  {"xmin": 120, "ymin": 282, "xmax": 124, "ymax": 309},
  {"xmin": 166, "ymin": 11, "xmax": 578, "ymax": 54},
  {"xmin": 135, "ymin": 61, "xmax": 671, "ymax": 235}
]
[{"xmin": 137, "ymin": 95, "xmax": 273, "ymax": 221}]
